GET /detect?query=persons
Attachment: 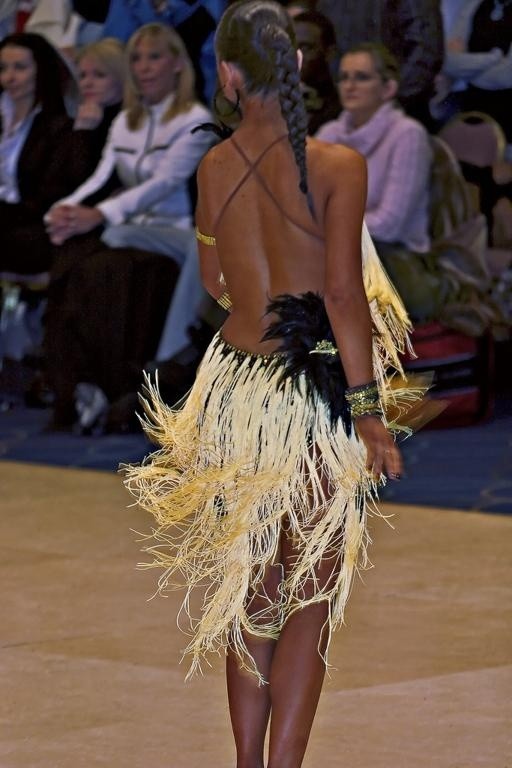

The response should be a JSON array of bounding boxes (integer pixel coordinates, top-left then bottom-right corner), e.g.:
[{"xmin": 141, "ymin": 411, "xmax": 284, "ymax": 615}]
[
  {"xmin": 117, "ymin": 1, "xmax": 429, "ymax": 767},
  {"xmin": 0, "ymin": 2, "xmax": 512, "ymax": 436}
]
